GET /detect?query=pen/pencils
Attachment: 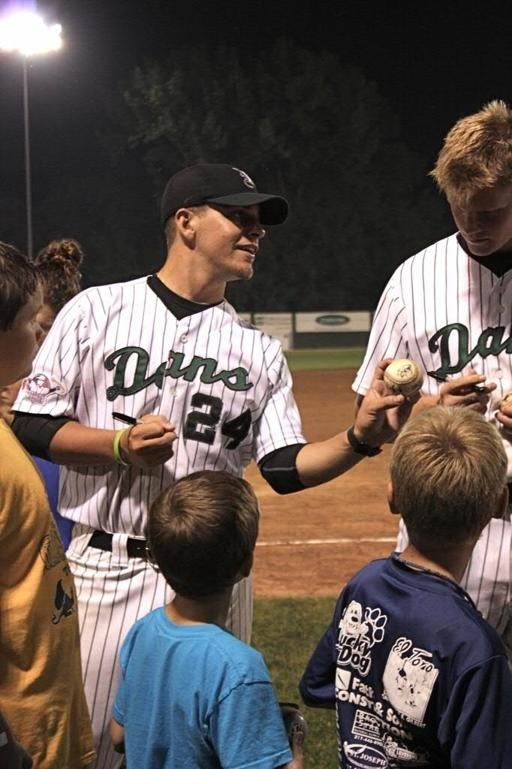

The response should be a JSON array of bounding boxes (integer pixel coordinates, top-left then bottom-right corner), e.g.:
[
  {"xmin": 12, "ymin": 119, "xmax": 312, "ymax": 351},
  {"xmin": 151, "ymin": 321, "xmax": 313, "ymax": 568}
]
[
  {"xmin": 427, "ymin": 372, "xmax": 484, "ymax": 392},
  {"xmin": 112, "ymin": 412, "xmax": 179, "ymax": 439}
]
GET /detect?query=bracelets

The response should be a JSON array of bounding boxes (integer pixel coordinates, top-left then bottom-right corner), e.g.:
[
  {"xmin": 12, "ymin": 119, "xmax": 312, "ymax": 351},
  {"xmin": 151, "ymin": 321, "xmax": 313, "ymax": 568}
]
[
  {"xmin": 347, "ymin": 426, "xmax": 383, "ymax": 457},
  {"xmin": 113, "ymin": 430, "xmax": 131, "ymax": 467}
]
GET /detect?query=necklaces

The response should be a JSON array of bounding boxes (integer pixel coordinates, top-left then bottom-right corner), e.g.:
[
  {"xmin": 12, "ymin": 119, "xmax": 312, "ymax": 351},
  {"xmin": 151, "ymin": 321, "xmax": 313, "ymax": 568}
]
[{"xmin": 391, "ymin": 551, "xmax": 477, "ymax": 609}]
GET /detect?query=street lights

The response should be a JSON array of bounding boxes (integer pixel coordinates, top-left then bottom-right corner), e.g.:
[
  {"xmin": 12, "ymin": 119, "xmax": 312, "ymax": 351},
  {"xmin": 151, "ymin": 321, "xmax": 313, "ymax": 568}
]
[{"xmin": 0, "ymin": 5, "xmax": 65, "ymax": 258}]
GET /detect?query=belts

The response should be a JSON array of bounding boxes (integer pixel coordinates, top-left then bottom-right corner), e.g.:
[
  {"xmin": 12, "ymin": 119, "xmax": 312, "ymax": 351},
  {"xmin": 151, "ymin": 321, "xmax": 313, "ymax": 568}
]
[
  {"xmin": 88, "ymin": 530, "xmax": 148, "ymax": 557},
  {"xmin": 507, "ymin": 483, "xmax": 511, "ymax": 503}
]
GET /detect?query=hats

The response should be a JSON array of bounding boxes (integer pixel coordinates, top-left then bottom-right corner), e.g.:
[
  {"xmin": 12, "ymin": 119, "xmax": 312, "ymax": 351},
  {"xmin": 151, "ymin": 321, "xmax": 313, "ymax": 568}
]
[{"xmin": 161, "ymin": 164, "xmax": 289, "ymax": 225}]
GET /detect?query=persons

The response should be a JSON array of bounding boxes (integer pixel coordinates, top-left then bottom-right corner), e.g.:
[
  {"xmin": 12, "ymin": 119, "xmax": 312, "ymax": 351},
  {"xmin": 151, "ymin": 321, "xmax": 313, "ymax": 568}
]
[
  {"xmin": 298, "ymin": 405, "xmax": 512, "ymax": 769},
  {"xmin": 351, "ymin": 100, "xmax": 511, "ymax": 653},
  {"xmin": 110, "ymin": 471, "xmax": 303, "ymax": 769},
  {"xmin": 1, "ymin": 242, "xmax": 97, "ymax": 769},
  {"xmin": 29, "ymin": 240, "xmax": 84, "ymax": 550},
  {"xmin": 2, "ymin": 162, "xmax": 419, "ymax": 769}
]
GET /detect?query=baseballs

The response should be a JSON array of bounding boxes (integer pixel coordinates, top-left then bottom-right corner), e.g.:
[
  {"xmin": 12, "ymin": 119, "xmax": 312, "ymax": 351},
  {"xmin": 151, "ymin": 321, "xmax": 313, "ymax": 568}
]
[{"xmin": 385, "ymin": 359, "xmax": 423, "ymax": 397}]
